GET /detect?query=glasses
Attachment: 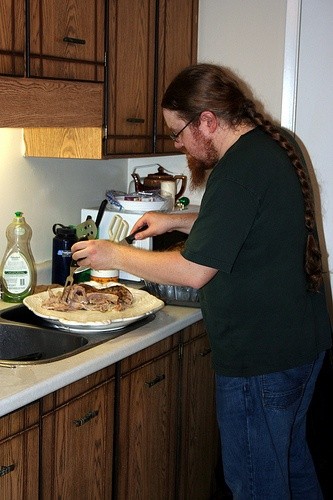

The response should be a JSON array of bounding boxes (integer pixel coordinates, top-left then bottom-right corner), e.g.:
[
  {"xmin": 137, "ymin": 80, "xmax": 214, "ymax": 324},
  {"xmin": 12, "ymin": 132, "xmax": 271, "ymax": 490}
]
[{"xmin": 170, "ymin": 115, "xmax": 199, "ymax": 142}]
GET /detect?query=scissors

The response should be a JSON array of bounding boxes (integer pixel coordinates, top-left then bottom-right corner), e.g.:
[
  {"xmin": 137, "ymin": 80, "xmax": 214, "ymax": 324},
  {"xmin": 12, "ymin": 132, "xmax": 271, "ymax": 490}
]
[{"xmin": 108, "ymin": 215, "xmax": 129, "ymax": 243}]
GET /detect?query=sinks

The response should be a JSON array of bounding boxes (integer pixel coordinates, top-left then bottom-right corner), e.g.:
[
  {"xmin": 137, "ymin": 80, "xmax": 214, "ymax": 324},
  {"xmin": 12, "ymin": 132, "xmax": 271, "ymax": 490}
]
[
  {"xmin": 0, "ymin": 320, "xmax": 92, "ymax": 365},
  {"xmin": 0, "ymin": 304, "xmax": 156, "ymax": 335}
]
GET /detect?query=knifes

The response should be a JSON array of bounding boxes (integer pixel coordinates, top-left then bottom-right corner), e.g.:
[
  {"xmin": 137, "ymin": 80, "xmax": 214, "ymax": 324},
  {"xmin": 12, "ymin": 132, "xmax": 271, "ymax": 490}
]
[{"xmin": 119, "ymin": 226, "xmax": 148, "ymax": 247}]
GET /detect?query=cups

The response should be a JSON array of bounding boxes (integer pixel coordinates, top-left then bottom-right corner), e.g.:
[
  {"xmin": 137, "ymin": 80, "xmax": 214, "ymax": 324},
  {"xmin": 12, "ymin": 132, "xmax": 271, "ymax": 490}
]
[{"xmin": 89, "ymin": 267, "xmax": 118, "ymax": 284}]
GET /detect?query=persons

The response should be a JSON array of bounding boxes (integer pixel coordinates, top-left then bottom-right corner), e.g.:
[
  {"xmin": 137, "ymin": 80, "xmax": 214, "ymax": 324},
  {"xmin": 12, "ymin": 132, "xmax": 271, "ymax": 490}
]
[{"xmin": 71, "ymin": 63, "xmax": 333, "ymax": 500}]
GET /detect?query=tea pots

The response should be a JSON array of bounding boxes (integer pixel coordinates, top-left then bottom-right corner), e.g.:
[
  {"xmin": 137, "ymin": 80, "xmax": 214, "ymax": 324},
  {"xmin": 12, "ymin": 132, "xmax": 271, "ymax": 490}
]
[{"xmin": 131, "ymin": 166, "xmax": 186, "ymax": 203}]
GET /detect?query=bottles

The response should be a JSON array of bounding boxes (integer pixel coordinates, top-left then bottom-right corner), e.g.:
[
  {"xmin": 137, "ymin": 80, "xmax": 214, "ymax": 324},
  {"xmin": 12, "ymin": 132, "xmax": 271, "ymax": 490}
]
[
  {"xmin": 0, "ymin": 211, "xmax": 37, "ymax": 303},
  {"xmin": 50, "ymin": 224, "xmax": 78, "ymax": 286}
]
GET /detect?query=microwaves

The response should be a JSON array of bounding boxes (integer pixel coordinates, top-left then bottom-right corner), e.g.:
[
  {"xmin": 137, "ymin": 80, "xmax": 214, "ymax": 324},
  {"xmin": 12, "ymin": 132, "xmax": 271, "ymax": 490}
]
[{"xmin": 80, "ymin": 205, "xmax": 202, "ymax": 282}]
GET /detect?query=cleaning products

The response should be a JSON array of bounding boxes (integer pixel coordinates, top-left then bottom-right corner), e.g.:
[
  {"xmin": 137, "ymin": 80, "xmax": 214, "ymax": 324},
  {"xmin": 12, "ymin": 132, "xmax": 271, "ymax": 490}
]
[{"xmin": 0, "ymin": 211, "xmax": 37, "ymax": 303}]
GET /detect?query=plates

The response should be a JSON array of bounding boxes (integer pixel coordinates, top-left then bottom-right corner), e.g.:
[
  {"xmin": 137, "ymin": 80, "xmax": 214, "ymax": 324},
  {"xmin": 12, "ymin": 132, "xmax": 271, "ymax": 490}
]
[
  {"xmin": 23, "ymin": 286, "xmax": 165, "ymax": 335},
  {"xmin": 115, "ymin": 196, "xmax": 167, "ymax": 213}
]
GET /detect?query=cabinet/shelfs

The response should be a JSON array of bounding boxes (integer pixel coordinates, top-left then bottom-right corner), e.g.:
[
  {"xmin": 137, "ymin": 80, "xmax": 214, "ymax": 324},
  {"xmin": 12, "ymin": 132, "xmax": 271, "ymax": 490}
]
[
  {"xmin": 0, "ymin": 279, "xmax": 228, "ymax": 499},
  {"xmin": 0, "ymin": 0, "xmax": 200, "ymax": 159}
]
